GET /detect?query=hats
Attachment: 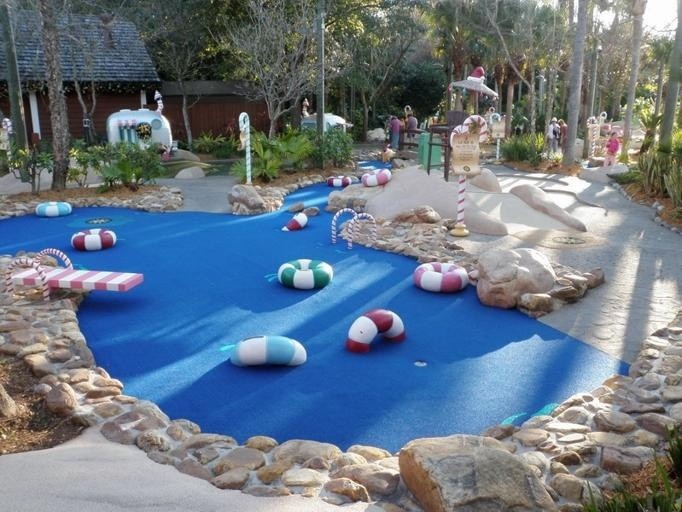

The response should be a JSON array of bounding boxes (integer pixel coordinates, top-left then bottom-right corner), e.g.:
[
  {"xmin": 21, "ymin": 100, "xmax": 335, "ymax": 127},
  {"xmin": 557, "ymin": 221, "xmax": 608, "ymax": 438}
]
[{"xmin": 551, "ymin": 117, "xmax": 557, "ymax": 122}]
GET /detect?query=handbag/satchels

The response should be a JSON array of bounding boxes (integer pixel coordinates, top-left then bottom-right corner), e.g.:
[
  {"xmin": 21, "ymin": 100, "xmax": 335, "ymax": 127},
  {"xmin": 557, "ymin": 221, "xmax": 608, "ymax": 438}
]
[{"xmin": 553, "ymin": 127, "xmax": 561, "ymax": 136}]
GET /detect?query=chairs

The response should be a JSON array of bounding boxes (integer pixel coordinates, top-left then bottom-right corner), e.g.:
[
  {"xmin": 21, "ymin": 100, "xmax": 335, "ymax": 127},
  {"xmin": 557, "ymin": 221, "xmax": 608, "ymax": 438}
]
[{"xmin": 428, "ymin": 110, "xmax": 470, "ymax": 182}]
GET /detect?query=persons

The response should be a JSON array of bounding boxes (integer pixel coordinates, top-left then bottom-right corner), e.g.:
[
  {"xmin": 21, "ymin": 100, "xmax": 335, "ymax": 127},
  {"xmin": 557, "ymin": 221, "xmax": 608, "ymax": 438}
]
[
  {"xmin": 172, "ymin": 122, "xmax": 186, "ymax": 139},
  {"xmin": 225, "ymin": 119, "xmax": 238, "ymax": 137},
  {"xmin": 389, "ymin": 112, "xmax": 397, "ymax": 146},
  {"xmin": 548, "ymin": 117, "xmax": 560, "ymax": 154},
  {"xmin": 407, "ymin": 114, "xmax": 418, "ymax": 149},
  {"xmin": 604, "ymin": 132, "xmax": 619, "ymax": 167},
  {"xmin": 390, "ymin": 117, "xmax": 403, "ymax": 149},
  {"xmin": 558, "ymin": 119, "xmax": 567, "ymax": 153}
]
